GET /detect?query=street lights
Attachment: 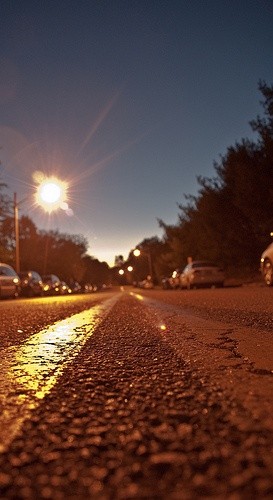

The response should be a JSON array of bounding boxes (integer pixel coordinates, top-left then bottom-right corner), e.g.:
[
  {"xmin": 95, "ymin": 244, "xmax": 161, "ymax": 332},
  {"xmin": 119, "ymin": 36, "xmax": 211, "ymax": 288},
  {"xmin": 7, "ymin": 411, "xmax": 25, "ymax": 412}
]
[
  {"xmin": 14, "ymin": 177, "xmax": 63, "ymax": 275},
  {"xmin": 133, "ymin": 249, "xmax": 153, "ymax": 278}
]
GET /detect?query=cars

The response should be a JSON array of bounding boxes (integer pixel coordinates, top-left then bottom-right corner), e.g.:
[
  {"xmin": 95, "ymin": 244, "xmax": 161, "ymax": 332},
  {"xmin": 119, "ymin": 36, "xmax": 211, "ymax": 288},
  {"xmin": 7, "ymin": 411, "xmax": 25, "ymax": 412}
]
[
  {"xmin": 19, "ymin": 269, "xmax": 44, "ymax": 297},
  {"xmin": 160, "ymin": 270, "xmax": 182, "ymax": 291},
  {"xmin": 0, "ymin": 262, "xmax": 21, "ymax": 299},
  {"xmin": 178, "ymin": 261, "xmax": 224, "ymax": 290},
  {"xmin": 132, "ymin": 278, "xmax": 154, "ymax": 289},
  {"xmin": 259, "ymin": 232, "xmax": 273, "ymax": 286},
  {"xmin": 60, "ymin": 281, "xmax": 113, "ymax": 296},
  {"xmin": 42, "ymin": 274, "xmax": 62, "ymax": 296}
]
